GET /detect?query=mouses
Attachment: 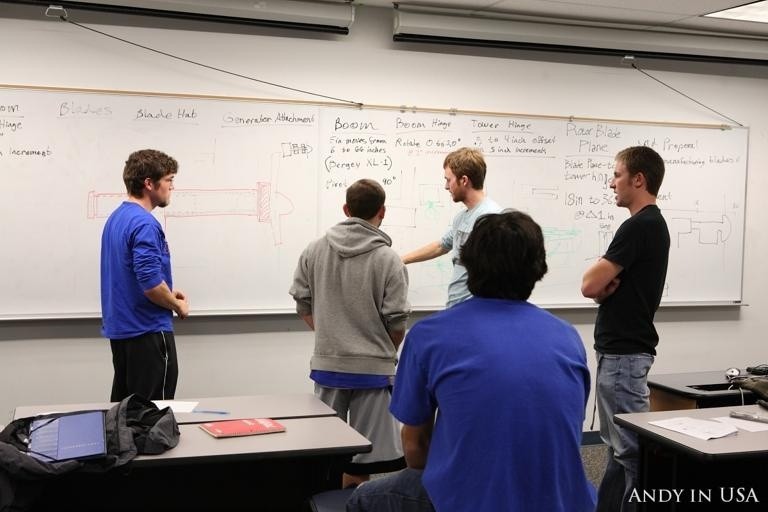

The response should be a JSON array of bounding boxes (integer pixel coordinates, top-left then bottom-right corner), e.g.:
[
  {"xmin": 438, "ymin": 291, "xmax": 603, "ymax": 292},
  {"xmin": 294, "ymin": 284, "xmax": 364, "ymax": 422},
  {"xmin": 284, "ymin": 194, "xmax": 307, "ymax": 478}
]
[{"xmin": 725, "ymin": 367, "xmax": 740, "ymax": 378}]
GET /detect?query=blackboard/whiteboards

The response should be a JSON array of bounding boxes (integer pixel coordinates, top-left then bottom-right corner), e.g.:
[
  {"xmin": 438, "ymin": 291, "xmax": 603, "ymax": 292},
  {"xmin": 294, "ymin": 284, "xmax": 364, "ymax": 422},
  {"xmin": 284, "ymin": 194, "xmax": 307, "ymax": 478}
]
[{"xmin": 0, "ymin": 83, "xmax": 750, "ymax": 321}]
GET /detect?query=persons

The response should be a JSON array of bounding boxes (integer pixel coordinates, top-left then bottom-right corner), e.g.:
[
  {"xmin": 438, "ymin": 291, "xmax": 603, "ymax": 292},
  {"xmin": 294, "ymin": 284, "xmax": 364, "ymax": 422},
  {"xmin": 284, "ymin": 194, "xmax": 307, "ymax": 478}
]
[
  {"xmin": 399, "ymin": 147, "xmax": 504, "ymax": 312},
  {"xmin": 347, "ymin": 208, "xmax": 599, "ymax": 512},
  {"xmin": 581, "ymin": 144, "xmax": 671, "ymax": 512},
  {"xmin": 289, "ymin": 179, "xmax": 409, "ymax": 490},
  {"xmin": 99, "ymin": 149, "xmax": 190, "ymax": 402}
]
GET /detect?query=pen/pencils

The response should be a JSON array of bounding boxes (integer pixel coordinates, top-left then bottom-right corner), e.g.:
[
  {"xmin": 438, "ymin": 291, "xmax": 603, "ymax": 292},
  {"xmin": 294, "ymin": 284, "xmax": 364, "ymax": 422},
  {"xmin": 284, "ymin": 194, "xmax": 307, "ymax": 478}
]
[{"xmin": 193, "ymin": 409, "xmax": 229, "ymax": 414}]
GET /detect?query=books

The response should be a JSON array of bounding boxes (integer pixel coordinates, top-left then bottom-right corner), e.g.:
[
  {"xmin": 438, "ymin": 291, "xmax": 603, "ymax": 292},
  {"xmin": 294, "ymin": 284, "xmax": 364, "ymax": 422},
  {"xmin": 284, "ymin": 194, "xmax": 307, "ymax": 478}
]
[
  {"xmin": 27, "ymin": 411, "xmax": 108, "ymax": 462},
  {"xmin": 199, "ymin": 418, "xmax": 287, "ymax": 438}
]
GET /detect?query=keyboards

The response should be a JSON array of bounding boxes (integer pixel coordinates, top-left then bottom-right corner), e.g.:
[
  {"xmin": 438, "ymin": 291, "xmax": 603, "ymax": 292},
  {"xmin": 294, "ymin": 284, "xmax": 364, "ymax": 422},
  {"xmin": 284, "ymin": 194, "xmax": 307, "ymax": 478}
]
[{"xmin": 746, "ymin": 366, "xmax": 768, "ymax": 375}]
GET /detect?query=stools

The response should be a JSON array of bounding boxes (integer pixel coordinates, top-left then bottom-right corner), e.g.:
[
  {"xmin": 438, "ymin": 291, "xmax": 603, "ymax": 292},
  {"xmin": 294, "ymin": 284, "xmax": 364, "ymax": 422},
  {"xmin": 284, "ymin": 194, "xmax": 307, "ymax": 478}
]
[
  {"xmin": 343, "ymin": 472, "xmax": 368, "ymax": 490},
  {"xmin": 309, "ymin": 489, "xmax": 354, "ymax": 512}
]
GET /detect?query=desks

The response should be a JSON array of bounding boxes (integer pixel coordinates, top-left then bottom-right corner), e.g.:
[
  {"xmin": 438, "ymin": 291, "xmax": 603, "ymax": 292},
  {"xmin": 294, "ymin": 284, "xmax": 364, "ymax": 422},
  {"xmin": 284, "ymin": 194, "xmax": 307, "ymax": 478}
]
[
  {"xmin": 12, "ymin": 415, "xmax": 372, "ymax": 512},
  {"xmin": 613, "ymin": 404, "xmax": 768, "ymax": 512},
  {"xmin": 15, "ymin": 396, "xmax": 338, "ymax": 422},
  {"xmin": 646, "ymin": 370, "xmax": 755, "ymax": 411}
]
